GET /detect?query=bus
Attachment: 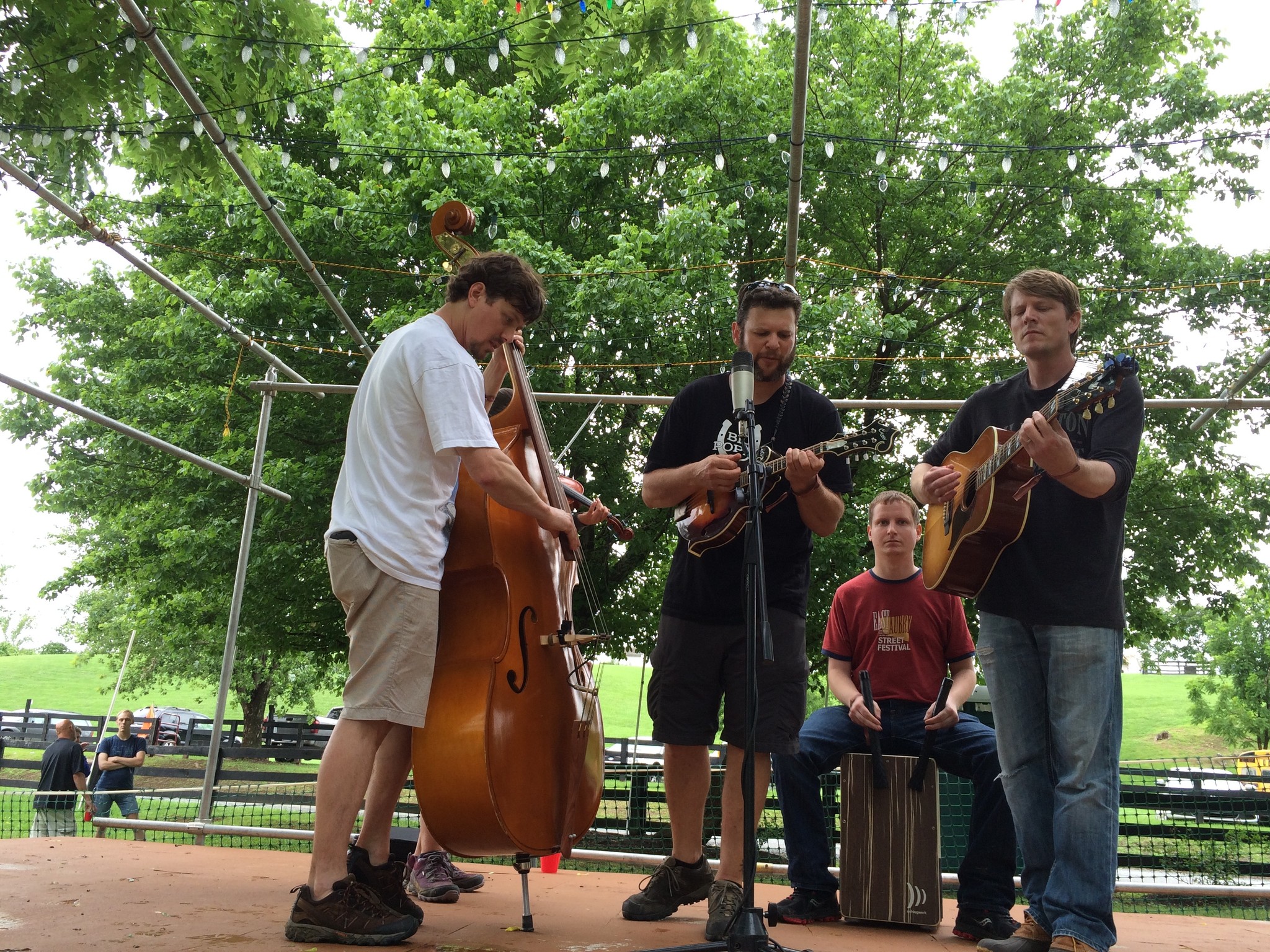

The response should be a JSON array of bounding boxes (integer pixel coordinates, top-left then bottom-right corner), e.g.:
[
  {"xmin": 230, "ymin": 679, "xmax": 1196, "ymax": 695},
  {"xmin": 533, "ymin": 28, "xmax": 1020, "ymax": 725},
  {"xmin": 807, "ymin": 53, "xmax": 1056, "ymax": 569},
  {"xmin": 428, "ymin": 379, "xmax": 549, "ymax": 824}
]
[{"xmin": 1235, "ymin": 750, "xmax": 1270, "ymax": 792}]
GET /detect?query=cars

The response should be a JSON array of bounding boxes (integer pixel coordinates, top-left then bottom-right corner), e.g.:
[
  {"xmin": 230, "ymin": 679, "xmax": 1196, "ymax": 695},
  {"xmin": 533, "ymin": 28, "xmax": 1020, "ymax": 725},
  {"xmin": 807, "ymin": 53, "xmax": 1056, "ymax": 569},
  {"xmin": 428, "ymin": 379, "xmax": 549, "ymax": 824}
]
[
  {"xmin": 0, "ymin": 707, "xmax": 94, "ymax": 745},
  {"xmin": 1151, "ymin": 766, "xmax": 1260, "ymax": 826},
  {"xmin": 604, "ymin": 734, "xmax": 666, "ymax": 783}
]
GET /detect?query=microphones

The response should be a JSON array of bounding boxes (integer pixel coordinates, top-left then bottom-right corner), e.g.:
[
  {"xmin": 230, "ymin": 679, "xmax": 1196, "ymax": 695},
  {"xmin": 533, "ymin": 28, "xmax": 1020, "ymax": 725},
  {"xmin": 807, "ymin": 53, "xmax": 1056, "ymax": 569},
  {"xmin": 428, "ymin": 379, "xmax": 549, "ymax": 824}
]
[{"xmin": 730, "ymin": 351, "xmax": 754, "ymax": 440}]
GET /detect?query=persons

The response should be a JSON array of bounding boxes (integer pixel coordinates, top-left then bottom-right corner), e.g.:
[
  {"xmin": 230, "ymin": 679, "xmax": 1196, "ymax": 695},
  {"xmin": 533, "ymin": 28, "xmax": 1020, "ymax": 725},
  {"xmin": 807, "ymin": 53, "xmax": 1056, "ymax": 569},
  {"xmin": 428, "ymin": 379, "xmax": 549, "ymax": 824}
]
[
  {"xmin": 403, "ymin": 386, "xmax": 609, "ymax": 902},
  {"xmin": 765, "ymin": 489, "xmax": 1022, "ymax": 944},
  {"xmin": 29, "ymin": 719, "xmax": 97, "ymax": 837},
  {"xmin": 610, "ymin": 280, "xmax": 854, "ymax": 939},
  {"xmin": 91, "ymin": 709, "xmax": 148, "ymax": 842},
  {"xmin": 911, "ymin": 267, "xmax": 1144, "ymax": 952},
  {"xmin": 286, "ymin": 252, "xmax": 579, "ymax": 944}
]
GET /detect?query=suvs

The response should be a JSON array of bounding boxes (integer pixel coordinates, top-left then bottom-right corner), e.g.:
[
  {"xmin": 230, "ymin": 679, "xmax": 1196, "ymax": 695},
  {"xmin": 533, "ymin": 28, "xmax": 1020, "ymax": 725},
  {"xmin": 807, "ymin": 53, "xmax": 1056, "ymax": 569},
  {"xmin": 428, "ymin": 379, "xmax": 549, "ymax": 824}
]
[{"xmin": 130, "ymin": 705, "xmax": 242, "ymax": 747}]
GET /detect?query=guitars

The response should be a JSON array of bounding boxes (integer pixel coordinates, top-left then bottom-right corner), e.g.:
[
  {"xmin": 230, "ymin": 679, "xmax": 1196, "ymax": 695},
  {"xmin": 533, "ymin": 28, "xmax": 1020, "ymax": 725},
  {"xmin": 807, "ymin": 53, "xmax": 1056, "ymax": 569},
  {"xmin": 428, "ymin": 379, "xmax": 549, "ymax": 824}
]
[
  {"xmin": 674, "ymin": 419, "xmax": 901, "ymax": 558},
  {"xmin": 921, "ymin": 349, "xmax": 1139, "ymax": 600}
]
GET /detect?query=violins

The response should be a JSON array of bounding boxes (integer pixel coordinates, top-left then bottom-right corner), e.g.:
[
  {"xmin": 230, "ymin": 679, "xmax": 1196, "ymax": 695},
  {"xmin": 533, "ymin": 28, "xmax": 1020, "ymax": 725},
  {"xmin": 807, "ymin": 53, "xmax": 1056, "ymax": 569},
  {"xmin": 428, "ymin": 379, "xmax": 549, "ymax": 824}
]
[{"xmin": 557, "ymin": 473, "xmax": 634, "ymax": 540}]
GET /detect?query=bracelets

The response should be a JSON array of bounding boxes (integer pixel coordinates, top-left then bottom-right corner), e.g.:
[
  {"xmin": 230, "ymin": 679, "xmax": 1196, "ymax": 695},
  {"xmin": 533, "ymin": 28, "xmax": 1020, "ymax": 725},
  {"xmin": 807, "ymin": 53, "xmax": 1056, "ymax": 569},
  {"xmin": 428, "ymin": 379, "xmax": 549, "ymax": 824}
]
[
  {"xmin": 850, "ymin": 694, "xmax": 863, "ymax": 708},
  {"xmin": 1045, "ymin": 459, "xmax": 1080, "ymax": 479},
  {"xmin": 791, "ymin": 473, "xmax": 820, "ymax": 498}
]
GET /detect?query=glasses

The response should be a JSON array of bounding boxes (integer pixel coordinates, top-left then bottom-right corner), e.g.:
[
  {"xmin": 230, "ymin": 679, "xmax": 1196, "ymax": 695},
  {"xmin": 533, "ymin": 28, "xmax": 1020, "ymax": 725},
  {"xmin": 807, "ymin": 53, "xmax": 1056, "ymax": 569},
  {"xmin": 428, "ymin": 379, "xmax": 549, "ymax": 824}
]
[{"xmin": 747, "ymin": 280, "xmax": 800, "ymax": 296}]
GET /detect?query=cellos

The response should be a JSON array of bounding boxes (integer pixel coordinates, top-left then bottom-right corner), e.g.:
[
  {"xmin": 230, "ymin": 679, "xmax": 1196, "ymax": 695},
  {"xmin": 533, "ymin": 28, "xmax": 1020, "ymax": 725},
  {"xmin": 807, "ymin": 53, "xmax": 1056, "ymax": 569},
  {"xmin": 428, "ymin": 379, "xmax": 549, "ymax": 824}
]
[{"xmin": 411, "ymin": 201, "xmax": 612, "ymax": 931}]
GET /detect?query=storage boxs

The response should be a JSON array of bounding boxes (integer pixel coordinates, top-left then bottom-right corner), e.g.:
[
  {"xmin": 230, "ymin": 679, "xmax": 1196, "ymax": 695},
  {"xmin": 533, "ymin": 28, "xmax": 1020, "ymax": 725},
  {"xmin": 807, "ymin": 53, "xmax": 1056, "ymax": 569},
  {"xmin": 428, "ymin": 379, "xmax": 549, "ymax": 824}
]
[{"xmin": 839, "ymin": 750, "xmax": 943, "ymax": 933}]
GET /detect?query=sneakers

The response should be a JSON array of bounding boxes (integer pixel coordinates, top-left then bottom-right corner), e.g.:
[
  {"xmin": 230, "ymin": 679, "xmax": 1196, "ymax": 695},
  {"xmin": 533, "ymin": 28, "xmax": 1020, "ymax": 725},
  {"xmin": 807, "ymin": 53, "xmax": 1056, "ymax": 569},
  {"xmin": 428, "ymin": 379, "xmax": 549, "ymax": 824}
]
[
  {"xmin": 285, "ymin": 871, "xmax": 420, "ymax": 946},
  {"xmin": 952, "ymin": 908, "xmax": 1021, "ymax": 941},
  {"xmin": 403, "ymin": 852, "xmax": 485, "ymax": 892},
  {"xmin": 706, "ymin": 878, "xmax": 742, "ymax": 942},
  {"xmin": 775, "ymin": 886, "xmax": 841, "ymax": 925},
  {"xmin": 345, "ymin": 842, "xmax": 425, "ymax": 926},
  {"xmin": 976, "ymin": 909, "xmax": 1052, "ymax": 952},
  {"xmin": 1048, "ymin": 933, "xmax": 1098, "ymax": 952},
  {"xmin": 407, "ymin": 853, "xmax": 460, "ymax": 903},
  {"xmin": 622, "ymin": 853, "xmax": 715, "ymax": 922}
]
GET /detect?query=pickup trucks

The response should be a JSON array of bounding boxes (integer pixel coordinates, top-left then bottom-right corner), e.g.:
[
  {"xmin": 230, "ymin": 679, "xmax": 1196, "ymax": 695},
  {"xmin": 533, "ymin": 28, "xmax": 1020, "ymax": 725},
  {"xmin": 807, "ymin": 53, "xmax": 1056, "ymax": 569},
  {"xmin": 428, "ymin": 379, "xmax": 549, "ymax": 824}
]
[{"xmin": 262, "ymin": 706, "xmax": 346, "ymax": 762}]
